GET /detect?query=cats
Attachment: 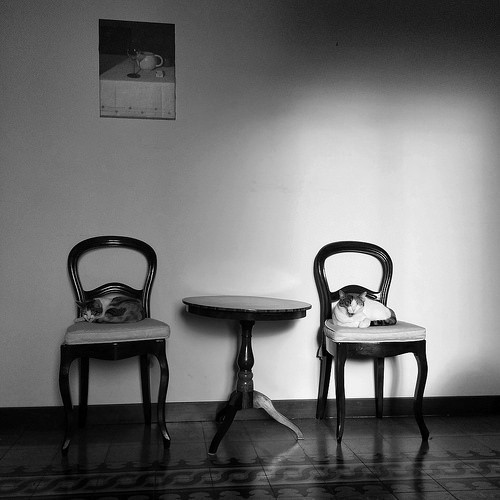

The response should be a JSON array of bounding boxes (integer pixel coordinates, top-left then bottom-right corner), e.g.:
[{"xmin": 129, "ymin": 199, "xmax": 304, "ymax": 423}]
[
  {"xmin": 73, "ymin": 295, "xmax": 146, "ymax": 324},
  {"xmin": 331, "ymin": 292, "xmax": 397, "ymax": 330}
]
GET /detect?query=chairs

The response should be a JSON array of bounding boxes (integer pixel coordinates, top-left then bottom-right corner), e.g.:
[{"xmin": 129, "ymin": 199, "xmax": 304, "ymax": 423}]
[
  {"xmin": 58, "ymin": 236, "xmax": 175, "ymax": 454},
  {"xmin": 314, "ymin": 241, "xmax": 430, "ymax": 446}
]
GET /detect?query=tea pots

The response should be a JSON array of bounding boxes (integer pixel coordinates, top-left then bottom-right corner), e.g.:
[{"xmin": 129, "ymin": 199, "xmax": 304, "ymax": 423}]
[{"xmin": 136, "ymin": 51, "xmax": 163, "ymax": 72}]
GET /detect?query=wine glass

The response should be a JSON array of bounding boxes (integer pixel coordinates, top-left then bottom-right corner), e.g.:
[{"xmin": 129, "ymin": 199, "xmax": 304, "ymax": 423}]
[{"xmin": 127, "ymin": 47, "xmax": 143, "ymax": 78}]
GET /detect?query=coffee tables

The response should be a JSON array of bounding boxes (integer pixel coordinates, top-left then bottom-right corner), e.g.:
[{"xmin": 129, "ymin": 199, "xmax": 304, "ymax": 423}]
[{"xmin": 183, "ymin": 295, "xmax": 313, "ymax": 458}]
